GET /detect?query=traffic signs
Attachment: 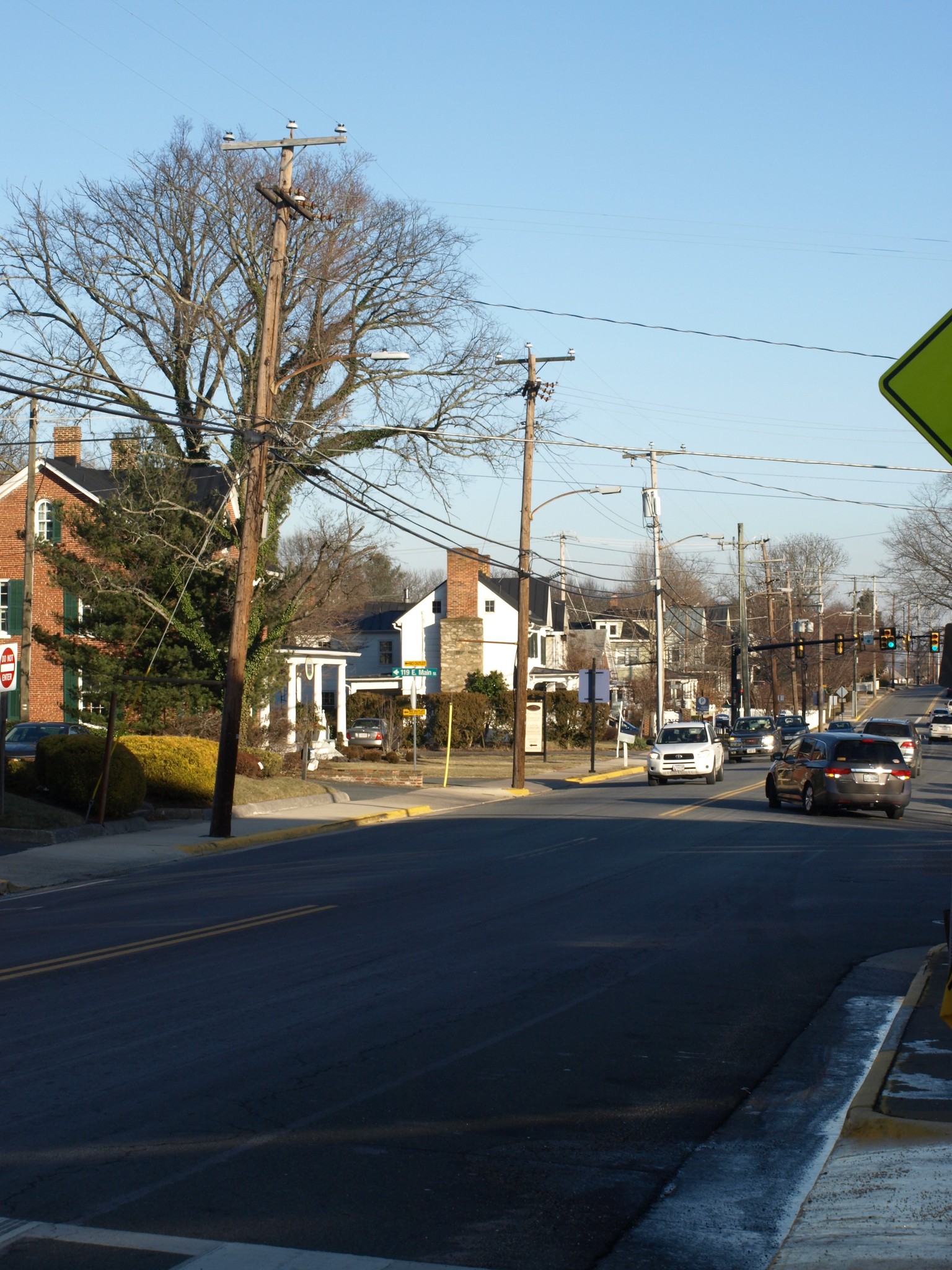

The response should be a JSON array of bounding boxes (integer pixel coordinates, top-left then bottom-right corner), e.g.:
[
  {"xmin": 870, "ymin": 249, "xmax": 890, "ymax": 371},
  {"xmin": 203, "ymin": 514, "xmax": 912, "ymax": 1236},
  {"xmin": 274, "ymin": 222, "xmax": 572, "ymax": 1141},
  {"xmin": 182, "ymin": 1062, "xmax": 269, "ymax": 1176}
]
[
  {"xmin": 392, "ymin": 668, "xmax": 437, "ymax": 677},
  {"xmin": 403, "ymin": 709, "xmax": 427, "ymax": 716},
  {"xmin": 404, "ymin": 661, "xmax": 427, "ymax": 666}
]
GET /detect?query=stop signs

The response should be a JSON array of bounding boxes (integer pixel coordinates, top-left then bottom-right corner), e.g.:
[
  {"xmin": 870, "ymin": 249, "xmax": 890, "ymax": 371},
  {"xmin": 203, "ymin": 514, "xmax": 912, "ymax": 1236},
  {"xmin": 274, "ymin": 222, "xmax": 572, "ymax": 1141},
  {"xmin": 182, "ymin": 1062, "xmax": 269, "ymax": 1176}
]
[{"xmin": 0, "ymin": 643, "xmax": 17, "ymax": 693}]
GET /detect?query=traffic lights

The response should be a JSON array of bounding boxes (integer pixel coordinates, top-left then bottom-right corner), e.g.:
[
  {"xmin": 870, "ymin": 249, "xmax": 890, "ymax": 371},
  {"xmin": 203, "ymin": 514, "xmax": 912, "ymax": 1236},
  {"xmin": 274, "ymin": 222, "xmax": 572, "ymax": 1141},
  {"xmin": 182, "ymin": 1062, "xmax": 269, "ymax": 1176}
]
[
  {"xmin": 930, "ymin": 631, "xmax": 940, "ymax": 653},
  {"xmin": 879, "ymin": 627, "xmax": 896, "ymax": 650},
  {"xmin": 902, "ymin": 633, "xmax": 910, "ymax": 653}
]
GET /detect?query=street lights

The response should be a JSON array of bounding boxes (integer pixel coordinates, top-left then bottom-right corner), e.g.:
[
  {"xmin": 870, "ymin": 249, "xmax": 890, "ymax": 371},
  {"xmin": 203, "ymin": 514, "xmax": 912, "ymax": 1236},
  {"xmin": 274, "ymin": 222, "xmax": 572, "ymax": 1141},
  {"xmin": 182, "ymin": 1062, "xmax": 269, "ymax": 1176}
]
[
  {"xmin": 818, "ymin": 609, "xmax": 855, "ymax": 733},
  {"xmin": 654, "ymin": 532, "xmax": 727, "ymax": 732},
  {"xmin": 740, "ymin": 587, "xmax": 794, "ymax": 715},
  {"xmin": 208, "ymin": 349, "xmax": 413, "ymax": 842},
  {"xmin": 511, "ymin": 484, "xmax": 622, "ymax": 791}
]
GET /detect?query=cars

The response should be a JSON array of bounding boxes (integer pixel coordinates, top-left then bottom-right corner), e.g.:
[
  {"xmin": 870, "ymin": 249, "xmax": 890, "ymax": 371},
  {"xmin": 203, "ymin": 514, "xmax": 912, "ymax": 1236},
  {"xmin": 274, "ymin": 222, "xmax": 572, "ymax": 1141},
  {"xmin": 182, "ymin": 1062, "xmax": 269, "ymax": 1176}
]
[
  {"xmin": 79, "ymin": 718, "xmax": 107, "ymax": 731},
  {"xmin": 4, "ymin": 721, "xmax": 92, "ymax": 790},
  {"xmin": 346, "ymin": 718, "xmax": 389, "ymax": 751},
  {"xmin": 825, "ymin": 721, "xmax": 856, "ymax": 733},
  {"xmin": 944, "ymin": 699, "xmax": 952, "ymax": 714}
]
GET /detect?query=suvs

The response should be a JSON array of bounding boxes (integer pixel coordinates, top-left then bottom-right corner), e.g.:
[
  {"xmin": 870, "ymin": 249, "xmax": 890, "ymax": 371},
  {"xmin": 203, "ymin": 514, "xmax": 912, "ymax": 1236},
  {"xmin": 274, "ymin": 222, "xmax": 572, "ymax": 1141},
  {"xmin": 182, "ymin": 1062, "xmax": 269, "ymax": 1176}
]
[
  {"xmin": 725, "ymin": 716, "xmax": 783, "ymax": 764},
  {"xmin": 764, "ymin": 732, "xmax": 913, "ymax": 819},
  {"xmin": 775, "ymin": 715, "xmax": 810, "ymax": 745},
  {"xmin": 643, "ymin": 721, "xmax": 725, "ymax": 787},
  {"xmin": 856, "ymin": 716, "xmax": 926, "ymax": 779},
  {"xmin": 926, "ymin": 714, "xmax": 952, "ymax": 741},
  {"xmin": 929, "ymin": 708, "xmax": 952, "ymax": 723}
]
